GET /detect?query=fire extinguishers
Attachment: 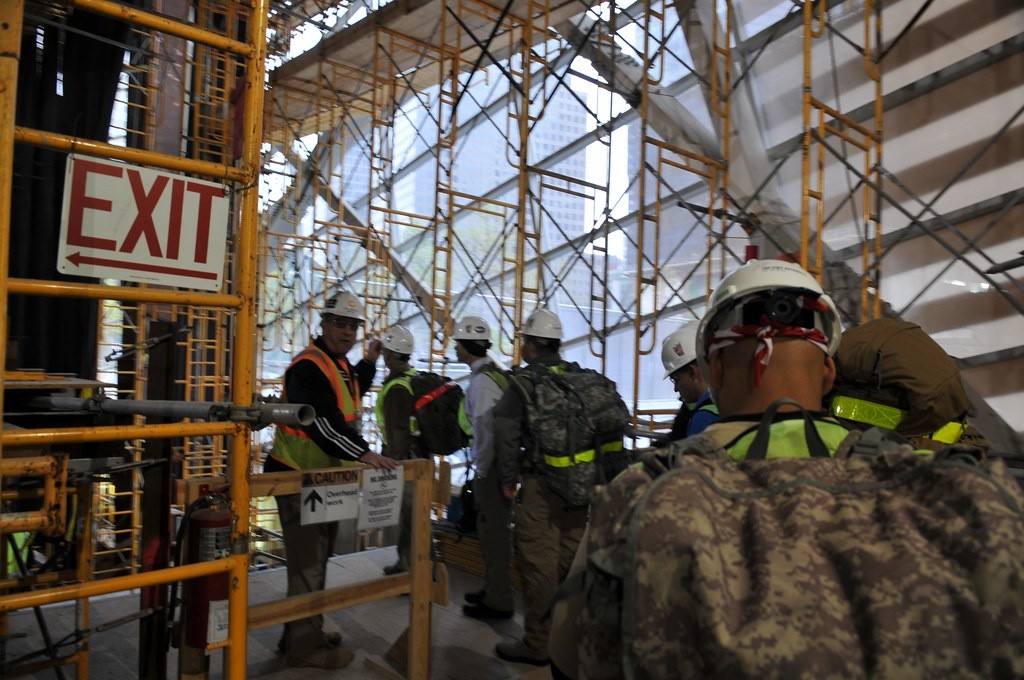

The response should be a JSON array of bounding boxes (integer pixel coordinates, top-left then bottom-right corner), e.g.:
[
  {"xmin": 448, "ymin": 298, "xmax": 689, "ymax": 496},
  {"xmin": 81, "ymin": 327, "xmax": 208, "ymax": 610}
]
[{"xmin": 164, "ymin": 483, "xmax": 231, "ymax": 649}]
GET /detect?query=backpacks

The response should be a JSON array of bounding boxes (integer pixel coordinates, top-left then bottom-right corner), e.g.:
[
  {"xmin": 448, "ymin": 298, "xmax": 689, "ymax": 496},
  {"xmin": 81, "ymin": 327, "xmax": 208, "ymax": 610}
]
[
  {"xmin": 833, "ymin": 317, "xmax": 978, "ymax": 438},
  {"xmin": 524, "ymin": 363, "xmax": 631, "ymax": 507},
  {"xmin": 620, "ymin": 429, "xmax": 1023, "ymax": 680},
  {"xmin": 404, "ymin": 370, "xmax": 469, "ymax": 456}
]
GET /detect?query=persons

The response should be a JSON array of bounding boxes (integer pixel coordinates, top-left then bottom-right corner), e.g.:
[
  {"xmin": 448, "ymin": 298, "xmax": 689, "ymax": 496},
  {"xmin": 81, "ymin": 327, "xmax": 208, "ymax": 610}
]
[
  {"xmin": 266, "ymin": 291, "xmax": 400, "ymax": 670},
  {"xmin": 448, "ymin": 316, "xmax": 517, "ymax": 622},
  {"xmin": 375, "ymin": 324, "xmax": 432, "ymax": 575},
  {"xmin": 548, "ymin": 259, "xmax": 1024, "ymax": 680},
  {"xmin": 493, "ymin": 307, "xmax": 587, "ymax": 666}
]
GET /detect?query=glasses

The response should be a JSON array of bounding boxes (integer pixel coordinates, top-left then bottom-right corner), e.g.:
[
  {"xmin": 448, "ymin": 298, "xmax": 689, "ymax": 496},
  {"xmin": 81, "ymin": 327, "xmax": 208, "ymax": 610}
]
[
  {"xmin": 669, "ymin": 377, "xmax": 677, "ymax": 385},
  {"xmin": 326, "ymin": 317, "xmax": 359, "ymax": 331}
]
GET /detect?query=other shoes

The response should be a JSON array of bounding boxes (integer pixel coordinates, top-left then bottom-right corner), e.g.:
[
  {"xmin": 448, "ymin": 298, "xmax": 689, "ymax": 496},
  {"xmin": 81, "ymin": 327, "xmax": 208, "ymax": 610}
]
[
  {"xmin": 462, "ymin": 590, "xmax": 515, "ymax": 621},
  {"xmin": 496, "ymin": 639, "xmax": 551, "ymax": 667},
  {"xmin": 278, "ymin": 631, "xmax": 354, "ymax": 670},
  {"xmin": 384, "ymin": 562, "xmax": 406, "ymax": 574}
]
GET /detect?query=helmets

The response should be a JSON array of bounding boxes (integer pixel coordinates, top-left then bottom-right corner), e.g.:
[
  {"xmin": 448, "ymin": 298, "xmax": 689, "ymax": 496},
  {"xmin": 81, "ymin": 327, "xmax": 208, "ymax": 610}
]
[
  {"xmin": 661, "ymin": 319, "xmax": 702, "ymax": 381},
  {"xmin": 452, "ymin": 316, "xmax": 490, "ymax": 341},
  {"xmin": 318, "ymin": 291, "xmax": 367, "ymax": 323},
  {"xmin": 695, "ymin": 258, "xmax": 841, "ymax": 387},
  {"xmin": 522, "ymin": 310, "xmax": 562, "ymax": 340},
  {"xmin": 380, "ymin": 324, "xmax": 414, "ymax": 355}
]
